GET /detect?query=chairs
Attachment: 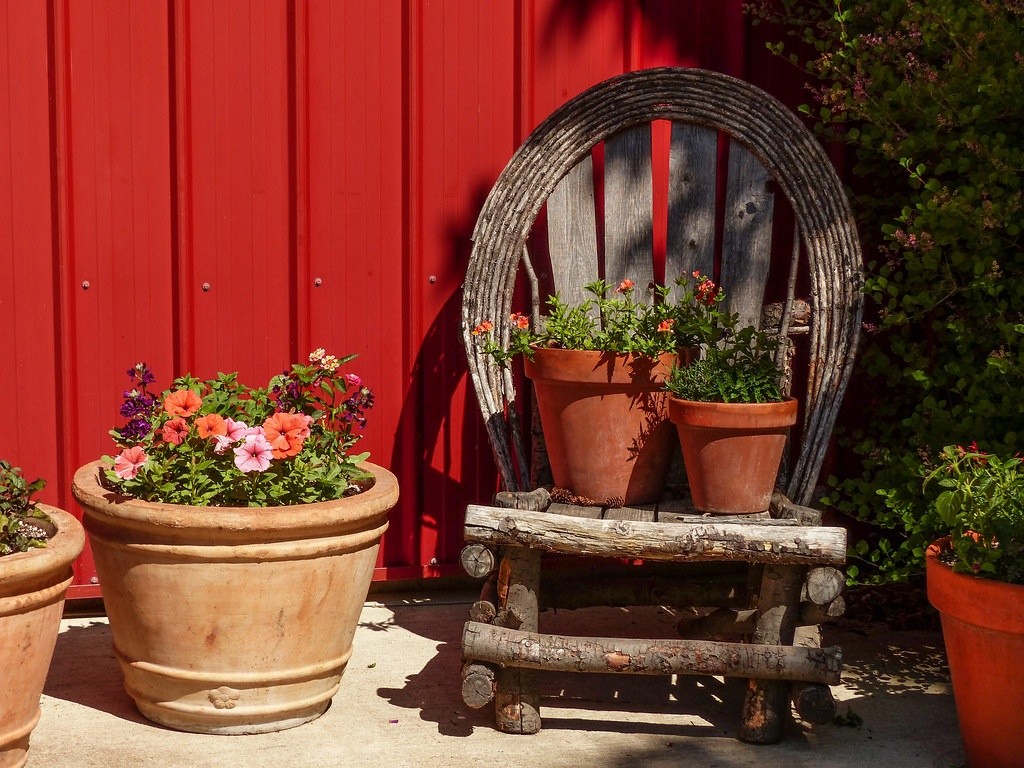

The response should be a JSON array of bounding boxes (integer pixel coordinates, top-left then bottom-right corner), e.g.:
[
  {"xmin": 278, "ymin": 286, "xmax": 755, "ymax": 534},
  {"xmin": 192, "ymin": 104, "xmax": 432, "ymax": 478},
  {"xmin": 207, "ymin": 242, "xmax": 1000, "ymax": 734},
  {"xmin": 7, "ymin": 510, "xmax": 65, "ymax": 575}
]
[{"xmin": 455, "ymin": 65, "xmax": 868, "ymax": 744}]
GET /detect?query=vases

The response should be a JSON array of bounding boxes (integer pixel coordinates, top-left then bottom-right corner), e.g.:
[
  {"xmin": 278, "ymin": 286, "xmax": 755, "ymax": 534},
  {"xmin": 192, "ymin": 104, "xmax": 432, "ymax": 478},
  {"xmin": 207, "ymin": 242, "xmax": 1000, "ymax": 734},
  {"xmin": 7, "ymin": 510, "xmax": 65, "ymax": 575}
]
[
  {"xmin": 71, "ymin": 451, "xmax": 401, "ymax": 735},
  {"xmin": 521, "ymin": 340, "xmax": 685, "ymax": 516},
  {"xmin": 668, "ymin": 394, "xmax": 798, "ymax": 515},
  {"xmin": 0, "ymin": 496, "xmax": 87, "ymax": 768},
  {"xmin": 925, "ymin": 528, "xmax": 1024, "ymax": 768}
]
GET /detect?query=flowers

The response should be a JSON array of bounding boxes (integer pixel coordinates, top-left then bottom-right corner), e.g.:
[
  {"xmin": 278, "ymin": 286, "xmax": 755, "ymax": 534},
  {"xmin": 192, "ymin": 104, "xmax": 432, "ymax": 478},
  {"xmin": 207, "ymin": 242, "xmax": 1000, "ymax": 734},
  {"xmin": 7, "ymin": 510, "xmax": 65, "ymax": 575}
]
[
  {"xmin": 84, "ymin": 341, "xmax": 393, "ymax": 506},
  {"xmin": 460, "ymin": 247, "xmax": 726, "ymax": 357},
  {"xmin": 926, "ymin": 439, "xmax": 1024, "ymax": 576}
]
